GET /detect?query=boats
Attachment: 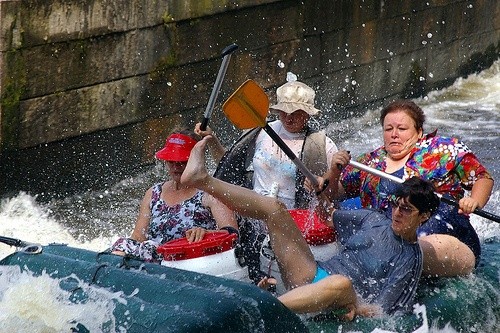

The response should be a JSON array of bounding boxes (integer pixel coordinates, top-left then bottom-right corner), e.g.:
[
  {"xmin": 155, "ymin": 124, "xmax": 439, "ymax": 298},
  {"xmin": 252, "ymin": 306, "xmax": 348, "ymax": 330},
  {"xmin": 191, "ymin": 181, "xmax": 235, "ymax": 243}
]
[{"xmin": 0, "ymin": 234, "xmax": 500, "ymax": 333}]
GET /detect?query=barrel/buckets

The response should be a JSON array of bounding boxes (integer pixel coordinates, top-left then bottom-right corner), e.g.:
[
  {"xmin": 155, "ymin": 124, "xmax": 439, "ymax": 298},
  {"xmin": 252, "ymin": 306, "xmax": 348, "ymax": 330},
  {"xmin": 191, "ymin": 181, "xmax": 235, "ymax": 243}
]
[
  {"xmin": 161, "ymin": 232, "xmax": 250, "ymax": 284},
  {"xmin": 260, "ymin": 210, "xmax": 342, "ymax": 318}
]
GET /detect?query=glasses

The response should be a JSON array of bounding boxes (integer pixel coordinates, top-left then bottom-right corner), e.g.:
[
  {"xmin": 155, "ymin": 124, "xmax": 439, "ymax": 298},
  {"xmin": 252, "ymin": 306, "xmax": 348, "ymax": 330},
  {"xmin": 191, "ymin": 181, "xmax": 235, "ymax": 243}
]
[{"xmin": 392, "ymin": 200, "xmax": 417, "ymax": 215}]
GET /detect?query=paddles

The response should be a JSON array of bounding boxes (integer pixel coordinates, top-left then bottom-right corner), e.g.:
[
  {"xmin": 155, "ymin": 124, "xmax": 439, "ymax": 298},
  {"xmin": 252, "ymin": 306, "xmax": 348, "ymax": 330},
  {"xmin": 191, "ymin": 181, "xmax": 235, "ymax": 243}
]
[
  {"xmin": 200, "ymin": 43, "xmax": 238, "ymax": 132},
  {"xmin": 221, "ymin": 79, "xmax": 329, "ymax": 196},
  {"xmin": 337, "ymin": 151, "xmax": 500, "ymax": 224}
]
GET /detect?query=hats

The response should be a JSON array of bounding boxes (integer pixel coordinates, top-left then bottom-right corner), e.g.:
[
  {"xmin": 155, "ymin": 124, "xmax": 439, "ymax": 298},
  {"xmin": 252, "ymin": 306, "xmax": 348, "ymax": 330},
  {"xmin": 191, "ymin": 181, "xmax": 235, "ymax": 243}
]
[
  {"xmin": 155, "ymin": 134, "xmax": 197, "ymax": 161},
  {"xmin": 269, "ymin": 81, "xmax": 322, "ymax": 116}
]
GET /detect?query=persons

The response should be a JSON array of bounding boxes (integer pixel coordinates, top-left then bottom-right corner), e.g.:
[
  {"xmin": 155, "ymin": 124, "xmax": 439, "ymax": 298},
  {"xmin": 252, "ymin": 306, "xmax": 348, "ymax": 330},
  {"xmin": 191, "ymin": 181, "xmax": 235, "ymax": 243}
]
[
  {"xmin": 194, "ymin": 81, "xmax": 339, "ymax": 292},
  {"xmin": 320, "ymin": 100, "xmax": 494, "ymax": 277},
  {"xmin": 107, "ymin": 134, "xmax": 239, "ymax": 264},
  {"xmin": 181, "ymin": 135, "xmax": 440, "ymax": 323}
]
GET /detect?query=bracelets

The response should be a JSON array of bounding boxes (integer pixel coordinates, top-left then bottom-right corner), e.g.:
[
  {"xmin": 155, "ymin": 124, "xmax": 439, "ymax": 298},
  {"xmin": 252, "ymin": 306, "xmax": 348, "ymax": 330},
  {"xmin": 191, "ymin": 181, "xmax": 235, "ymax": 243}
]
[{"xmin": 309, "ymin": 178, "xmax": 329, "ymax": 197}]
